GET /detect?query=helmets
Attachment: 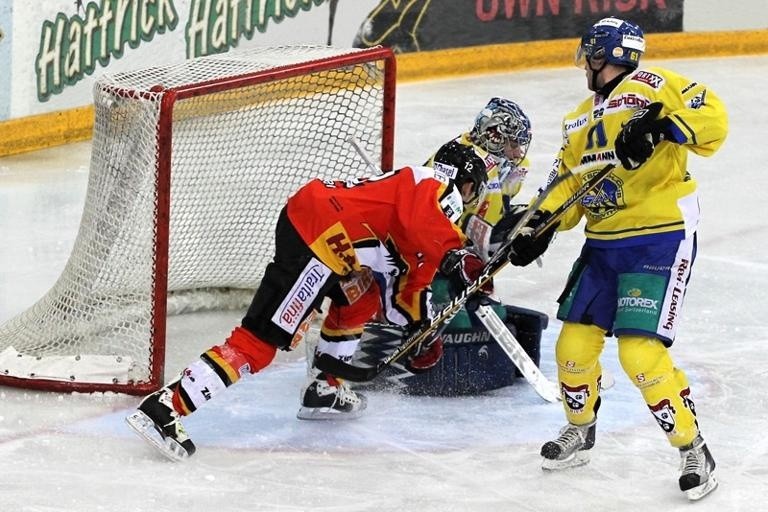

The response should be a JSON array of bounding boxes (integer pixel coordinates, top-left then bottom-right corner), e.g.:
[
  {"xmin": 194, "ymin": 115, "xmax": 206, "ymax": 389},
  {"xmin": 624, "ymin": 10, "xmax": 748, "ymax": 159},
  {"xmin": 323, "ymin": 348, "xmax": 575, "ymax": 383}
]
[
  {"xmin": 433, "ymin": 138, "xmax": 488, "ymax": 192},
  {"xmin": 581, "ymin": 16, "xmax": 646, "ymax": 68},
  {"xmin": 474, "ymin": 96, "xmax": 531, "ymax": 145}
]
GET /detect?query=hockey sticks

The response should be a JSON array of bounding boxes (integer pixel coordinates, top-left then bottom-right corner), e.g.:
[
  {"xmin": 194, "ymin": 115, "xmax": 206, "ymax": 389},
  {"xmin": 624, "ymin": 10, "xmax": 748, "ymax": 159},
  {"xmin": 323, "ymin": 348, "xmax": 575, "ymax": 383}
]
[
  {"xmin": 315, "ymin": 159, "xmax": 624, "ymax": 382},
  {"xmin": 422, "ymin": 178, "xmax": 565, "ymax": 348},
  {"xmin": 314, "ymin": 135, "xmax": 620, "ymax": 403},
  {"xmin": 348, "ymin": 135, "xmax": 616, "ymax": 403}
]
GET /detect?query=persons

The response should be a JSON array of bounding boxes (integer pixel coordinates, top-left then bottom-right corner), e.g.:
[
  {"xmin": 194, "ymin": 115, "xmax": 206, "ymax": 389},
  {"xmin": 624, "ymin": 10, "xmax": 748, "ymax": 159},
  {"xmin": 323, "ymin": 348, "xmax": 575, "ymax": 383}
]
[
  {"xmin": 505, "ymin": 18, "xmax": 732, "ymax": 499},
  {"xmin": 122, "ymin": 143, "xmax": 496, "ymax": 468},
  {"xmin": 307, "ymin": 97, "xmax": 549, "ymax": 398}
]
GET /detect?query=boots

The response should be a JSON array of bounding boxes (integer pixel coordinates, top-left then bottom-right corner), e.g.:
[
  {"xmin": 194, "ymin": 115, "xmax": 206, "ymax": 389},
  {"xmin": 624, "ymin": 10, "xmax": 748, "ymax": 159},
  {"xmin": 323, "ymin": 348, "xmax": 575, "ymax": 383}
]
[
  {"xmin": 137, "ymin": 379, "xmax": 196, "ymax": 454},
  {"xmin": 540, "ymin": 423, "xmax": 596, "ymax": 460},
  {"xmin": 679, "ymin": 434, "xmax": 716, "ymax": 492},
  {"xmin": 302, "ymin": 379, "xmax": 368, "ymax": 413}
]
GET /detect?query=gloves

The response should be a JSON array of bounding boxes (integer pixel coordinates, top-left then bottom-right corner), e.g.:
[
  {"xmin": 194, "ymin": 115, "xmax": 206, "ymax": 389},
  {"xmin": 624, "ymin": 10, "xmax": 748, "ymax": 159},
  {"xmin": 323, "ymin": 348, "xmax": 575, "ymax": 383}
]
[
  {"xmin": 615, "ymin": 102, "xmax": 663, "ymax": 171},
  {"xmin": 444, "ymin": 248, "xmax": 495, "ymax": 313},
  {"xmin": 490, "ymin": 203, "xmax": 563, "ymax": 268},
  {"xmin": 404, "ymin": 318, "xmax": 444, "ymax": 374}
]
